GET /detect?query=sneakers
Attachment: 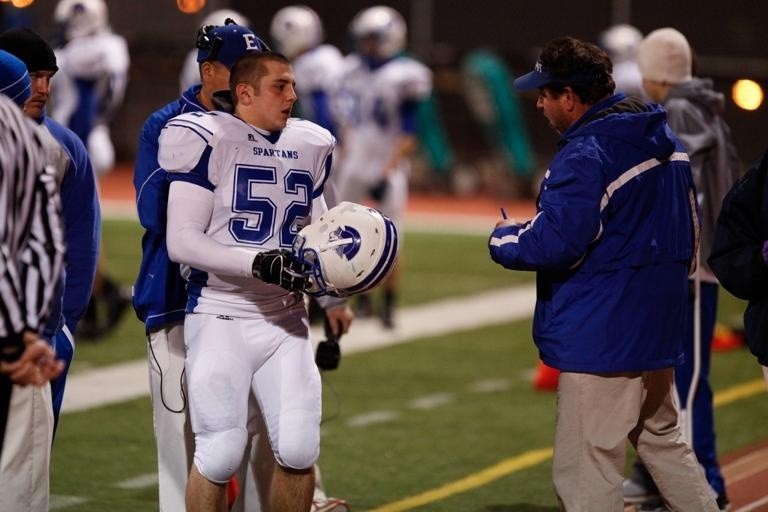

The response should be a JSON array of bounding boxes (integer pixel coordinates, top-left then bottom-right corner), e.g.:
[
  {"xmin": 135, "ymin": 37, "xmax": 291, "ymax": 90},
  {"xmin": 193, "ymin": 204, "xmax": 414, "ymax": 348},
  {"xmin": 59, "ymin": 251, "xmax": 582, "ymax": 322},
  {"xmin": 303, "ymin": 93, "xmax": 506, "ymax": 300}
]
[
  {"xmin": 310, "ymin": 488, "xmax": 350, "ymax": 511},
  {"xmin": 620, "ymin": 461, "xmax": 661, "ymax": 505}
]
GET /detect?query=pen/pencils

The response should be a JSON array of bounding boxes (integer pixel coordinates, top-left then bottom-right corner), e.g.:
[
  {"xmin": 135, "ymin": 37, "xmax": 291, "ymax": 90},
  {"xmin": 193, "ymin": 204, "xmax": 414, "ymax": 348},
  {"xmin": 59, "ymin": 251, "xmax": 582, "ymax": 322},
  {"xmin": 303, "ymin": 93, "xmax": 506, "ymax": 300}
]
[{"xmin": 501, "ymin": 208, "xmax": 507, "ymax": 220}]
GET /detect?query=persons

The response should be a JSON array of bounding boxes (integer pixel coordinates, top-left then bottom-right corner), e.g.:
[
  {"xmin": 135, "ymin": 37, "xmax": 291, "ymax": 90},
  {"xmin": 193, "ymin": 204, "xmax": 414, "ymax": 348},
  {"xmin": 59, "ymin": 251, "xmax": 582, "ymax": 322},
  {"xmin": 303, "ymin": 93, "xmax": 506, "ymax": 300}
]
[
  {"xmin": 487, "ymin": 24, "xmax": 767, "ymax": 512},
  {"xmin": 0, "ymin": 2, "xmax": 484, "ymax": 511}
]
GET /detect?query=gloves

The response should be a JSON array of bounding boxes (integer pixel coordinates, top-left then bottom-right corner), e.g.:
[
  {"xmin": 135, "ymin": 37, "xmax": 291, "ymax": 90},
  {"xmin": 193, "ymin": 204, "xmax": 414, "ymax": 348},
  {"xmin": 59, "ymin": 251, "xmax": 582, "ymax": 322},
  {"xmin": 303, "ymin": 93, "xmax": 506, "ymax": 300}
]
[{"xmin": 250, "ymin": 247, "xmax": 315, "ymax": 293}]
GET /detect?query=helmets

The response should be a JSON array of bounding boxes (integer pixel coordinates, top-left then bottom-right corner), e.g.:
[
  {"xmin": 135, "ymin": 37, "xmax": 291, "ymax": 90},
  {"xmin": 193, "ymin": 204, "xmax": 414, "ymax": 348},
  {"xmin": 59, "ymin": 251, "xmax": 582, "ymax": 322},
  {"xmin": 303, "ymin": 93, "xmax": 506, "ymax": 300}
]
[{"xmin": 289, "ymin": 198, "xmax": 400, "ymax": 301}]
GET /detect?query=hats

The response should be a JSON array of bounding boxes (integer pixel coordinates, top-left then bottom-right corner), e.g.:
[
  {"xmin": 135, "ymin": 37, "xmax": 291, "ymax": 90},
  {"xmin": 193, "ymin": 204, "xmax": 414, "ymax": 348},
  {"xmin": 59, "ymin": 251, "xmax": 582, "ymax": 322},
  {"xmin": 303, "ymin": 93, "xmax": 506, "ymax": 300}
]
[
  {"xmin": 636, "ymin": 25, "xmax": 694, "ymax": 86},
  {"xmin": 512, "ymin": 52, "xmax": 613, "ymax": 92},
  {"xmin": 193, "ymin": 23, "xmax": 263, "ymax": 77},
  {"xmin": 1, "ymin": 25, "xmax": 60, "ymax": 74},
  {"xmin": 0, "ymin": 47, "xmax": 32, "ymax": 107}
]
